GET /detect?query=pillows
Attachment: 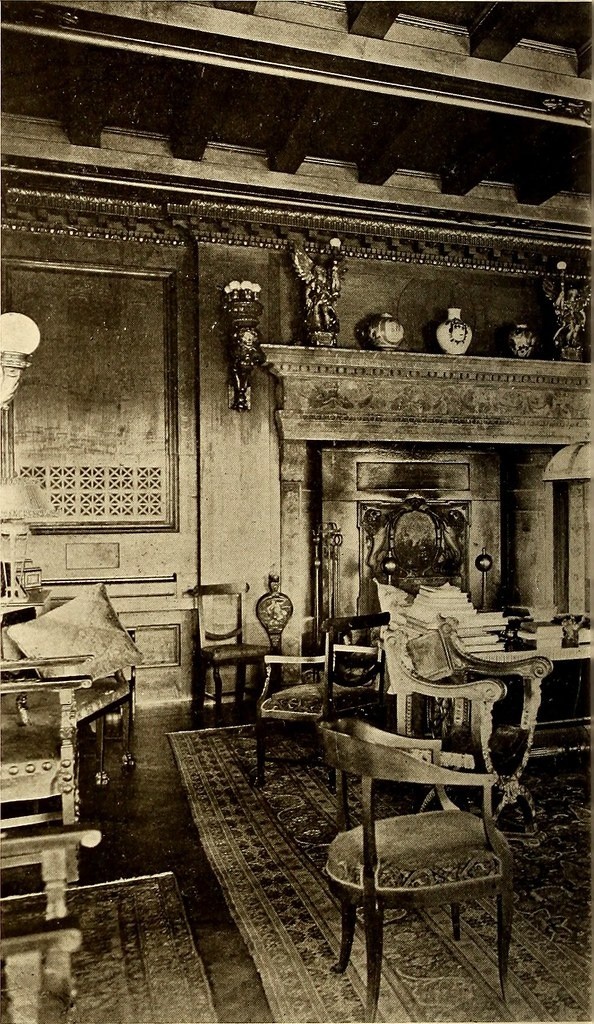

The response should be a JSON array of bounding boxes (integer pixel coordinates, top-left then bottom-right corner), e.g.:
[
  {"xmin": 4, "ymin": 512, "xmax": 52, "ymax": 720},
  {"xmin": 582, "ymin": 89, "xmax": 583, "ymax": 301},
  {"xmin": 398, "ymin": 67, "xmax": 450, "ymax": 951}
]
[{"xmin": 6, "ymin": 583, "xmax": 144, "ymax": 696}]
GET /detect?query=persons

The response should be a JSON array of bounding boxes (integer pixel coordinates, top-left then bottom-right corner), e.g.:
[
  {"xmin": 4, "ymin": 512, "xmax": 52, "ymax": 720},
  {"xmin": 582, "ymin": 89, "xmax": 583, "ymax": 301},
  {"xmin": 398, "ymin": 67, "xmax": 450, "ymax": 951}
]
[
  {"xmin": 556, "ymin": 289, "xmax": 584, "ymax": 349},
  {"xmin": 304, "ymin": 265, "xmax": 333, "ymax": 331}
]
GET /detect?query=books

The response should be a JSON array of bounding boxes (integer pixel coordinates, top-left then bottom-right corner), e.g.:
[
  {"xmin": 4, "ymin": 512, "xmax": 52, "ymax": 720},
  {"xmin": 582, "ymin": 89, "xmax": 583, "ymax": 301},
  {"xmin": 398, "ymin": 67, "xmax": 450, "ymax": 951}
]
[{"xmin": 380, "ymin": 578, "xmax": 593, "ymax": 656}]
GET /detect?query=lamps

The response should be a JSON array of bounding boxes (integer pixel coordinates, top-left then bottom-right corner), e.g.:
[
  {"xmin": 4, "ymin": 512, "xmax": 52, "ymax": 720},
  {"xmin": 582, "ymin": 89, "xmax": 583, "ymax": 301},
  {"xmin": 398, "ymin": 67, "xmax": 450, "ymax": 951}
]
[
  {"xmin": 0, "ymin": 312, "xmax": 40, "ymax": 412},
  {"xmin": 0, "ymin": 477, "xmax": 58, "ymax": 601}
]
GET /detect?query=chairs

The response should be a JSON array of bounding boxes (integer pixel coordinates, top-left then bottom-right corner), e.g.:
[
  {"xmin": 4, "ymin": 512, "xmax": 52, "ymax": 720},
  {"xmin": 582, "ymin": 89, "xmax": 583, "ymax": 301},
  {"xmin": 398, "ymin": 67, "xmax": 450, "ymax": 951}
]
[
  {"xmin": 0, "ymin": 602, "xmax": 219, "ymax": 1024},
  {"xmin": 318, "ymin": 719, "xmax": 517, "ymax": 1024},
  {"xmin": 190, "ymin": 580, "xmax": 553, "ymax": 834}
]
